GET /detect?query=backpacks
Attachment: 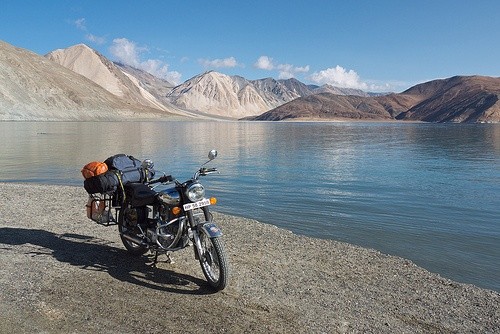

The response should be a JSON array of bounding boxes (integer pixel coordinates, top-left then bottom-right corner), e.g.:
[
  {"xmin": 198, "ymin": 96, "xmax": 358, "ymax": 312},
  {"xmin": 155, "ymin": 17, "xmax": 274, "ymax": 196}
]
[{"xmin": 104, "ymin": 154, "xmax": 143, "ymax": 169}]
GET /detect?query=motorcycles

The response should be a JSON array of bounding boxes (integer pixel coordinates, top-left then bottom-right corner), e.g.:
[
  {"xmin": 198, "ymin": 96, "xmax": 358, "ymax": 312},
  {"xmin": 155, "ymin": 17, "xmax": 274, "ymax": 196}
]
[{"xmin": 80, "ymin": 149, "xmax": 230, "ymax": 291}]
